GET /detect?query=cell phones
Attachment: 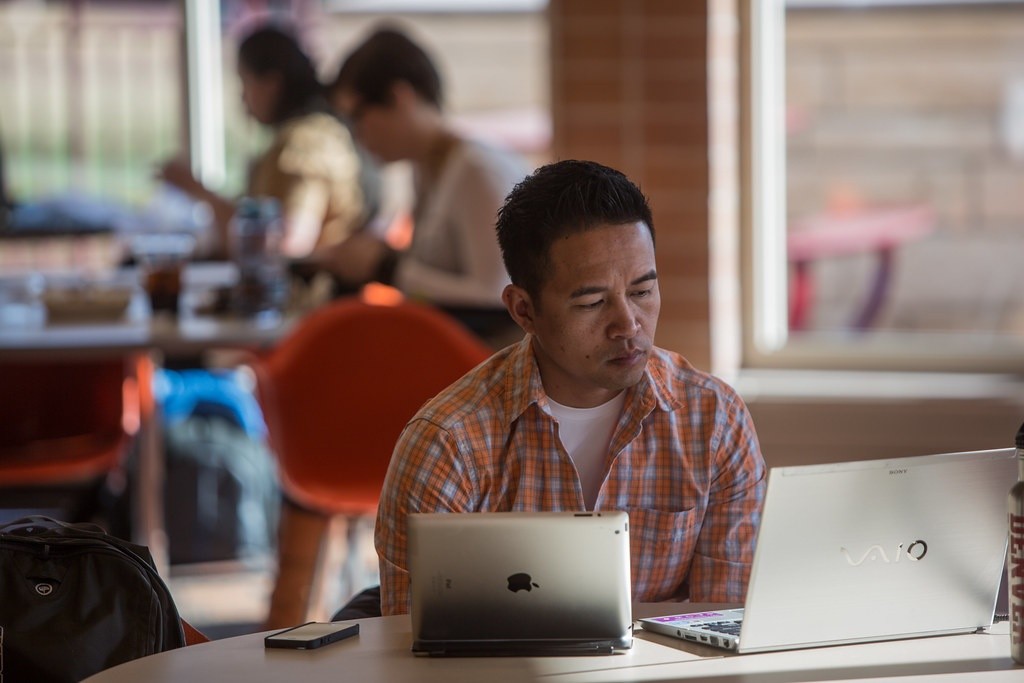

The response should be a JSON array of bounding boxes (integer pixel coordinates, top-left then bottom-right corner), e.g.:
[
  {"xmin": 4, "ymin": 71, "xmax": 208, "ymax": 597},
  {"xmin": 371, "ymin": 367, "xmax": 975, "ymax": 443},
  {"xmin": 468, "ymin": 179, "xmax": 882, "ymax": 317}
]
[{"xmin": 264, "ymin": 621, "xmax": 360, "ymax": 650}]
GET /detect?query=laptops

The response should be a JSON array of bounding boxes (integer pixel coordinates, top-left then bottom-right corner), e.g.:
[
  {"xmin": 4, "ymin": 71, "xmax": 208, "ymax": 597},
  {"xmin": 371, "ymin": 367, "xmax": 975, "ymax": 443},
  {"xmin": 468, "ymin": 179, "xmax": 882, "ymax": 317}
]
[{"xmin": 638, "ymin": 448, "xmax": 1024, "ymax": 653}]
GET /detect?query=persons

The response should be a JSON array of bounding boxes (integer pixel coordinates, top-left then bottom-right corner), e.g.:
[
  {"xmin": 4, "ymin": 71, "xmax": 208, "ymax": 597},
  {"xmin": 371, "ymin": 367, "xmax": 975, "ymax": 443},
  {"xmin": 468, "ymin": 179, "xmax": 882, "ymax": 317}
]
[
  {"xmin": 375, "ymin": 160, "xmax": 765, "ymax": 615},
  {"xmin": 70, "ymin": 20, "xmax": 515, "ymax": 527}
]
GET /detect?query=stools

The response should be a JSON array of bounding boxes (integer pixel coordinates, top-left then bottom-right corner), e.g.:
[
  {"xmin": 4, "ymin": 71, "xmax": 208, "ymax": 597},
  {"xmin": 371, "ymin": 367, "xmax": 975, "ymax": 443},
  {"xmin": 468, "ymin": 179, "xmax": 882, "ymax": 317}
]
[{"xmin": 156, "ymin": 369, "xmax": 269, "ymax": 558}]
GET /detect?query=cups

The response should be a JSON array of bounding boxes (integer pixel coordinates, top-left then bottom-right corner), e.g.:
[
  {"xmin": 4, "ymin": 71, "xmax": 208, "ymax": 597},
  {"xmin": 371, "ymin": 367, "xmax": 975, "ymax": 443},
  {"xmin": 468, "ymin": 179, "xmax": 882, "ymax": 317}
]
[{"xmin": 134, "ymin": 237, "xmax": 194, "ymax": 315}]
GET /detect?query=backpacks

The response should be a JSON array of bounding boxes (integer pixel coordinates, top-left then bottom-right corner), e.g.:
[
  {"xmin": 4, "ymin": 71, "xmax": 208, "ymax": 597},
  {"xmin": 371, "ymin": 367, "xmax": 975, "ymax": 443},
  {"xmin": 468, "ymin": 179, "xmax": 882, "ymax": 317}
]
[{"xmin": 0, "ymin": 515, "xmax": 186, "ymax": 683}]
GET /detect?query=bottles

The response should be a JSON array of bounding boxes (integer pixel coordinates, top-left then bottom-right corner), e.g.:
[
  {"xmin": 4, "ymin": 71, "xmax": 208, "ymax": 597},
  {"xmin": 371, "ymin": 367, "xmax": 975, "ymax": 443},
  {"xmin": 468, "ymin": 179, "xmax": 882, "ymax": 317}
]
[
  {"xmin": 1006, "ymin": 420, "xmax": 1023, "ymax": 667},
  {"xmin": 228, "ymin": 198, "xmax": 286, "ymax": 328}
]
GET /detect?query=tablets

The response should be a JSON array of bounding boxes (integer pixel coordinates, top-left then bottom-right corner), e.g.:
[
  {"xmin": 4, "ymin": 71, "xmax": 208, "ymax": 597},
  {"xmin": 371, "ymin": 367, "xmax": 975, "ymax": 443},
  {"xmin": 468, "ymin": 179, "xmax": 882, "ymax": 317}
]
[{"xmin": 406, "ymin": 510, "xmax": 634, "ymax": 655}]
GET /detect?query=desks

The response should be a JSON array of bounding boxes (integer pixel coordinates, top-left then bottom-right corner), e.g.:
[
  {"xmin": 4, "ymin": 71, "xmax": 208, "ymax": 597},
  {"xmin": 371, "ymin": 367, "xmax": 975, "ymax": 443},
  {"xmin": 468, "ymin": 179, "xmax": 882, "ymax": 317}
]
[
  {"xmin": 79, "ymin": 603, "xmax": 1024, "ymax": 683},
  {"xmin": 0, "ymin": 266, "xmax": 276, "ymax": 587}
]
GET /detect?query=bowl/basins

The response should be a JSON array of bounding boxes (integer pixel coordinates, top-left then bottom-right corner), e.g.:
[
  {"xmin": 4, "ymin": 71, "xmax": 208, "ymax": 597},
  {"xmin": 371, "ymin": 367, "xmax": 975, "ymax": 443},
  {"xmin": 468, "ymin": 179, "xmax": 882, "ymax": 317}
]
[{"xmin": 40, "ymin": 287, "xmax": 129, "ymax": 321}]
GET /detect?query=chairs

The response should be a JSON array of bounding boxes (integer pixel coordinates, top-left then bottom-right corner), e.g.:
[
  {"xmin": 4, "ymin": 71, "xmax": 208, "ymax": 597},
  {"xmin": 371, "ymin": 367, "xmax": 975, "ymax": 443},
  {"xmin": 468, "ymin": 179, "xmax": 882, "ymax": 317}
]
[
  {"xmin": 0, "ymin": 349, "xmax": 141, "ymax": 488},
  {"xmin": 257, "ymin": 293, "xmax": 489, "ymax": 629}
]
